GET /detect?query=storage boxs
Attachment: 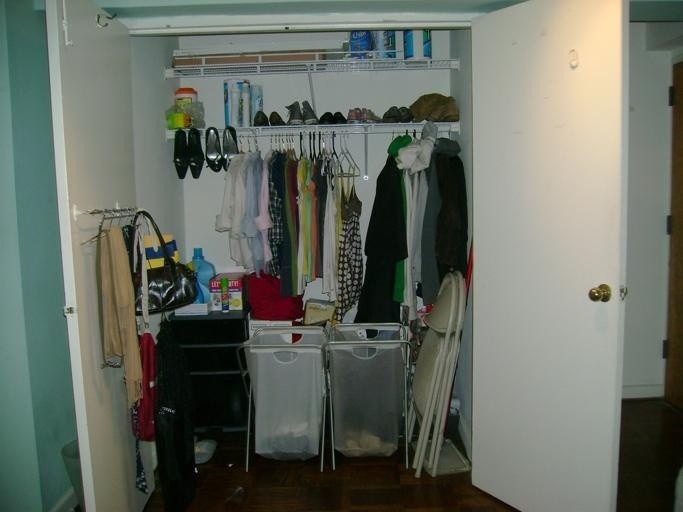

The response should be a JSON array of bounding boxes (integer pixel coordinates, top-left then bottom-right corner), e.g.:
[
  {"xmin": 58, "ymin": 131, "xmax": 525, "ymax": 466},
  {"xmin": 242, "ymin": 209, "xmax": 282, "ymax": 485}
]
[
  {"xmin": 208, "ymin": 270, "xmax": 247, "ymax": 312},
  {"xmin": 248, "ymin": 317, "xmax": 292, "ymax": 345}
]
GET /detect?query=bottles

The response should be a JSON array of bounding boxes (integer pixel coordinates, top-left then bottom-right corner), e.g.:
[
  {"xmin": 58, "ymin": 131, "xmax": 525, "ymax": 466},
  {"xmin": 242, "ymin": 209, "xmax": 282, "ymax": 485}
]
[
  {"xmin": 230, "ymin": 82, "xmax": 264, "ymax": 129},
  {"xmin": 341, "ymin": 32, "xmax": 351, "ymax": 59},
  {"xmin": 174, "ymin": 88, "xmax": 198, "ymax": 106}
]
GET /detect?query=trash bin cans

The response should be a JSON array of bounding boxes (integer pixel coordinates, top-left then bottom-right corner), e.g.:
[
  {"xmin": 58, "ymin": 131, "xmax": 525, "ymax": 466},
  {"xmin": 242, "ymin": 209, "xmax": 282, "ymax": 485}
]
[
  {"xmin": 238, "ymin": 322, "xmax": 411, "ymax": 472},
  {"xmin": 61, "ymin": 440, "xmax": 84, "ymax": 512}
]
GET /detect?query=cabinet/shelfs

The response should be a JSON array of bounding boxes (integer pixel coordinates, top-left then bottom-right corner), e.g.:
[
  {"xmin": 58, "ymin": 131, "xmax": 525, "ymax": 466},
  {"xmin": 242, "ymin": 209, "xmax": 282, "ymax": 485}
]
[
  {"xmin": 165, "ymin": 309, "xmax": 251, "ymax": 449},
  {"xmin": 41, "ymin": 0, "xmax": 637, "ymax": 512}
]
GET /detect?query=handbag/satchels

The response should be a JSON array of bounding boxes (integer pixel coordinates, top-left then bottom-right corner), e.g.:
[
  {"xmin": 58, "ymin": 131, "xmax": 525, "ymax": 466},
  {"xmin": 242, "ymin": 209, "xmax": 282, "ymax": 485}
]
[
  {"xmin": 119, "ymin": 210, "xmax": 199, "ymax": 318},
  {"xmin": 130, "ymin": 326, "xmax": 158, "ymax": 442}
]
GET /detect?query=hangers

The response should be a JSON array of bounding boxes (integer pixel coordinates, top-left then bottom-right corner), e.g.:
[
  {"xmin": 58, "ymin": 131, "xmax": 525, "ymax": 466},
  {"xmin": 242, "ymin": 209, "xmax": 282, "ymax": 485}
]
[
  {"xmin": 236, "ymin": 128, "xmax": 364, "ymax": 179},
  {"xmin": 385, "ymin": 125, "xmax": 455, "ymax": 144},
  {"xmin": 80, "ymin": 206, "xmax": 135, "ymax": 246}
]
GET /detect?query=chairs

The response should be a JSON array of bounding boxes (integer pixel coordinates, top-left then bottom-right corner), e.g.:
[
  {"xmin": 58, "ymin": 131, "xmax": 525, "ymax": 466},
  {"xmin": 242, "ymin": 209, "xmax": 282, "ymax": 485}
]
[{"xmin": 405, "ymin": 268, "xmax": 466, "ymax": 481}]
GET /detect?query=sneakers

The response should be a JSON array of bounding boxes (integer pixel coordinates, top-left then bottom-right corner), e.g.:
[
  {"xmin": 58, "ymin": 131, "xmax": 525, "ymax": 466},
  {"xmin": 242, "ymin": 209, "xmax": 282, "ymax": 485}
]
[{"xmin": 252, "ymin": 91, "xmax": 460, "ymax": 128}]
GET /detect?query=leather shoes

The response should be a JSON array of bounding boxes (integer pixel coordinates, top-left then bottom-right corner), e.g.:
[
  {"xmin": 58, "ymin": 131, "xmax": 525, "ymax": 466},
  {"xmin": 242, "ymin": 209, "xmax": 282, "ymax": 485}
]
[
  {"xmin": 187, "ymin": 129, "xmax": 205, "ymax": 180},
  {"xmin": 224, "ymin": 125, "xmax": 240, "ymax": 174},
  {"xmin": 172, "ymin": 128, "xmax": 190, "ymax": 180},
  {"xmin": 205, "ymin": 125, "xmax": 222, "ymax": 174}
]
